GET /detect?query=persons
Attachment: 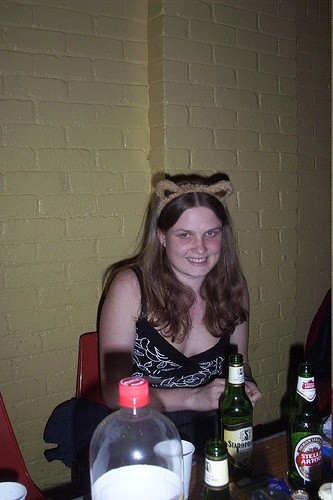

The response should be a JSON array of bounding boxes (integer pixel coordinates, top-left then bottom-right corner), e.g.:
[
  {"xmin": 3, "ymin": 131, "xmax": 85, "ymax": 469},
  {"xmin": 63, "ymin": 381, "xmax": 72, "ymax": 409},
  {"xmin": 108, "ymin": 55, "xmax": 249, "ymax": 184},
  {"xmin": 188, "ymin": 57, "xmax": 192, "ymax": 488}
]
[{"xmin": 99, "ymin": 179, "xmax": 262, "ymax": 466}]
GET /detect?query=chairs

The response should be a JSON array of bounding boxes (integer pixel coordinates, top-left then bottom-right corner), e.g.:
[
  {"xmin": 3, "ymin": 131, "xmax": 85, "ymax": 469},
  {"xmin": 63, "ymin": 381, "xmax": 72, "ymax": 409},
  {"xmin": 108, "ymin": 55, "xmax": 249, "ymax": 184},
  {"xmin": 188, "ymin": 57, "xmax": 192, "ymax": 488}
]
[{"xmin": 75, "ymin": 332, "xmax": 105, "ymax": 406}]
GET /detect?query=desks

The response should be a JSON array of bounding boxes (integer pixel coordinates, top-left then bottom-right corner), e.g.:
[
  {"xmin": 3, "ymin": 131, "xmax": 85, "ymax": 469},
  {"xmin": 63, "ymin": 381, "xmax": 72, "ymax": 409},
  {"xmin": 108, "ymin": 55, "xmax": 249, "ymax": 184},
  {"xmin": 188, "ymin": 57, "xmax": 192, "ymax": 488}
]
[{"xmin": 75, "ymin": 413, "xmax": 333, "ymax": 500}]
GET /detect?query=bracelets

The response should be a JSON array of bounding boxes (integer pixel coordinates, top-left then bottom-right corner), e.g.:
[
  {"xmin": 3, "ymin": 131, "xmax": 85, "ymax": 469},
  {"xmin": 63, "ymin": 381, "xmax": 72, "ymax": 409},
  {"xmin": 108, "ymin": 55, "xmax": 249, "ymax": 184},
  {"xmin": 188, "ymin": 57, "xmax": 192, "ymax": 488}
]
[{"xmin": 244, "ymin": 374, "xmax": 257, "ymax": 384}]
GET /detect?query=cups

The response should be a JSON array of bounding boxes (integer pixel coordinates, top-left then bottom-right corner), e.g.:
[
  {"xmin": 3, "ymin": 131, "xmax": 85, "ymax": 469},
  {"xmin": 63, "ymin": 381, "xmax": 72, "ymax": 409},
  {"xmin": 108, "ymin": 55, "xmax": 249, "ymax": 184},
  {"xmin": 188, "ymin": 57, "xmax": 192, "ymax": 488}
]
[
  {"xmin": 0, "ymin": 481, "xmax": 27, "ymax": 500},
  {"xmin": 153, "ymin": 439, "xmax": 194, "ymax": 500}
]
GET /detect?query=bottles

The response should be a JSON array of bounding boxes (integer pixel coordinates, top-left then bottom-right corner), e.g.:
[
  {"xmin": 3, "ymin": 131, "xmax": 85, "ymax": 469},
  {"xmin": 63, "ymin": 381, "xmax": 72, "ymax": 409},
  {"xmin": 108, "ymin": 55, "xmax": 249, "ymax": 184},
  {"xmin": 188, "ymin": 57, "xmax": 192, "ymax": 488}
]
[
  {"xmin": 202, "ymin": 439, "xmax": 234, "ymax": 500},
  {"xmin": 88, "ymin": 378, "xmax": 183, "ymax": 500},
  {"xmin": 217, "ymin": 352, "xmax": 255, "ymax": 479},
  {"xmin": 285, "ymin": 360, "xmax": 324, "ymax": 491}
]
